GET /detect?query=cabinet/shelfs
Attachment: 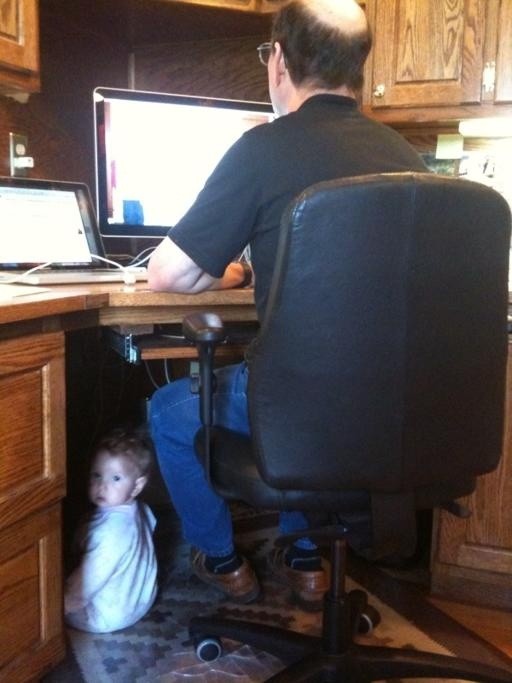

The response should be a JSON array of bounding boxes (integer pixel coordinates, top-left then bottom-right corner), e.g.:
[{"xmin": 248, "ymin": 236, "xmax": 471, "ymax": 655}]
[
  {"xmin": 0, "ymin": 332, "xmax": 73, "ymax": 683},
  {"xmin": 0, "ymin": 0, "xmax": 47, "ymax": 106},
  {"xmin": 428, "ymin": 342, "xmax": 512, "ymax": 614},
  {"xmin": 360, "ymin": 1, "xmax": 511, "ymax": 129}
]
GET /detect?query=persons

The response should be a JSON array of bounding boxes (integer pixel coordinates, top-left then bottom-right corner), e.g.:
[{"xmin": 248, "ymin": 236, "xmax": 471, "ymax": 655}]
[
  {"xmin": 147, "ymin": 1, "xmax": 438, "ymax": 604},
  {"xmin": 64, "ymin": 423, "xmax": 158, "ymax": 632}
]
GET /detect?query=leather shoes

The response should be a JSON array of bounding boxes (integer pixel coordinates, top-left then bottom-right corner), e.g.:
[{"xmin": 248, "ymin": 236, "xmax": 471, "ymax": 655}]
[
  {"xmin": 266, "ymin": 537, "xmax": 330, "ymax": 602},
  {"xmin": 189, "ymin": 544, "xmax": 260, "ymax": 604}
]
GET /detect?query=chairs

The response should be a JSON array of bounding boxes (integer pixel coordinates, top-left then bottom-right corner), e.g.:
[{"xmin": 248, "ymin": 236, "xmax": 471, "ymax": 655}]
[{"xmin": 180, "ymin": 180, "xmax": 512, "ymax": 670}]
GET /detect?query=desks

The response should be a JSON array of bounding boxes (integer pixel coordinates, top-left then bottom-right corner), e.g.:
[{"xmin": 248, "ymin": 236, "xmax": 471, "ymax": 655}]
[{"xmin": 2, "ymin": 242, "xmax": 512, "ymax": 390}]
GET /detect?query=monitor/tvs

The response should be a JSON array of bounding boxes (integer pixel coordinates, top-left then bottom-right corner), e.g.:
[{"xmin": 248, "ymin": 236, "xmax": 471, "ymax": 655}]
[{"xmin": 92, "ymin": 88, "xmax": 275, "ymax": 239}]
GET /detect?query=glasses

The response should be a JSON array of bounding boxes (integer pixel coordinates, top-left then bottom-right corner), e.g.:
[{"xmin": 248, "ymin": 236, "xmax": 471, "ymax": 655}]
[{"xmin": 257, "ymin": 42, "xmax": 272, "ymax": 66}]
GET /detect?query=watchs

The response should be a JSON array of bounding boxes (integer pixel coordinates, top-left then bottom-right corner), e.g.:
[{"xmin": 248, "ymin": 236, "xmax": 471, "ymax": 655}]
[{"xmin": 233, "ymin": 262, "xmax": 253, "ymax": 289}]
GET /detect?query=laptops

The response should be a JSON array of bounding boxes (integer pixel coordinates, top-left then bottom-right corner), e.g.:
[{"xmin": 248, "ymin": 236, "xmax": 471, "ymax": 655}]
[{"xmin": 0, "ymin": 175, "xmax": 147, "ymax": 285}]
